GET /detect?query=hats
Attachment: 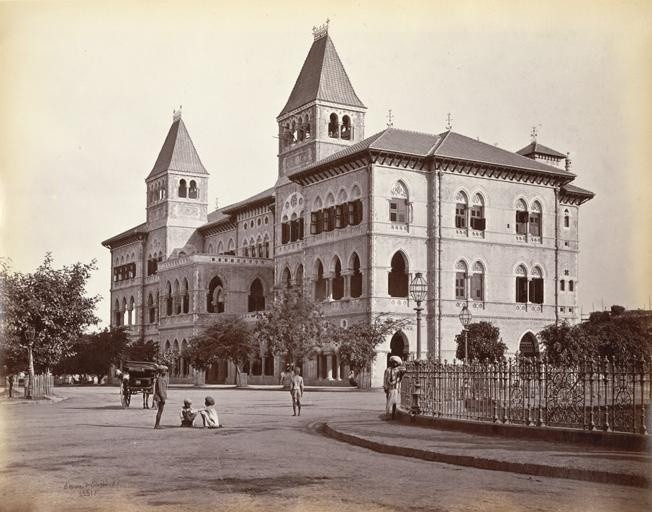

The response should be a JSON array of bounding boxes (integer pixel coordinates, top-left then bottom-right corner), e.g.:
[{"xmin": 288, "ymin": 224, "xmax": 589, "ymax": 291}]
[
  {"xmin": 294, "ymin": 367, "xmax": 300, "ymax": 373},
  {"xmin": 184, "ymin": 399, "xmax": 192, "ymax": 403},
  {"xmin": 390, "ymin": 356, "xmax": 401, "ymax": 364},
  {"xmin": 158, "ymin": 365, "xmax": 168, "ymax": 369},
  {"xmin": 206, "ymin": 397, "xmax": 214, "ymax": 405}
]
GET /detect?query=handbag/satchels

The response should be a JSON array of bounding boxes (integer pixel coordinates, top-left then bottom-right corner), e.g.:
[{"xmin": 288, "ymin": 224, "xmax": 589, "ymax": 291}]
[{"xmin": 389, "ymin": 388, "xmax": 401, "ymax": 403}]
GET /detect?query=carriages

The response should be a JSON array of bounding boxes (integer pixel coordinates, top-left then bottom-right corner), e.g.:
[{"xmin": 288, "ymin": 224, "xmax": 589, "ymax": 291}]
[{"xmin": 119, "ymin": 361, "xmax": 158, "ymax": 408}]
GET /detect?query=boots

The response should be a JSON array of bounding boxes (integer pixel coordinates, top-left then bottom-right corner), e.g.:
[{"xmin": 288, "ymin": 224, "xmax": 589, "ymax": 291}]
[
  {"xmin": 293, "ymin": 405, "xmax": 300, "ymax": 416},
  {"xmin": 155, "ymin": 414, "xmax": 161, "ymax": 428}
]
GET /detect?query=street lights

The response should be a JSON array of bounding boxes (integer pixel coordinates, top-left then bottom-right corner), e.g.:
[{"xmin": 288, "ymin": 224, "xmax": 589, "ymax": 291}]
[
  {"xmin": 409, "ymin": 272, "xmax": 428, "ymax": 412},
  {"xmin": 459, "ymin": 303, "xmax": 472, "ymax": 400},
  {"xmin": 25, "ymin": 327, "xmax": 35, "ymax": 398}
]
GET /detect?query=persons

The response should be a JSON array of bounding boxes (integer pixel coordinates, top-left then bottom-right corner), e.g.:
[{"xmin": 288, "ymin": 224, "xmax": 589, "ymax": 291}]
[
  {"xmin": 198, "ymin": 396, "xmax": 220, "ymax": 428},
  {"xmin": 8, "ymin": 374, "xmax": 15, "ymax": 397},
  {"xmin": 383, "ymin": 355, "xmax": 406, "ymax": 417},
  {"xmin": 153, "ymin": 364, "xmax": 170, "ymax": 428},
  {"xmin": 179, "ymin": 399, "xmax": 197, "ymax": 427},
  {"xmin": 22, "ymin": 371, "xmax": 30, "ymax": 399},
  {"xmin": 290, "ymin": 367, "xmax": 305, "ymax": 416}
]
